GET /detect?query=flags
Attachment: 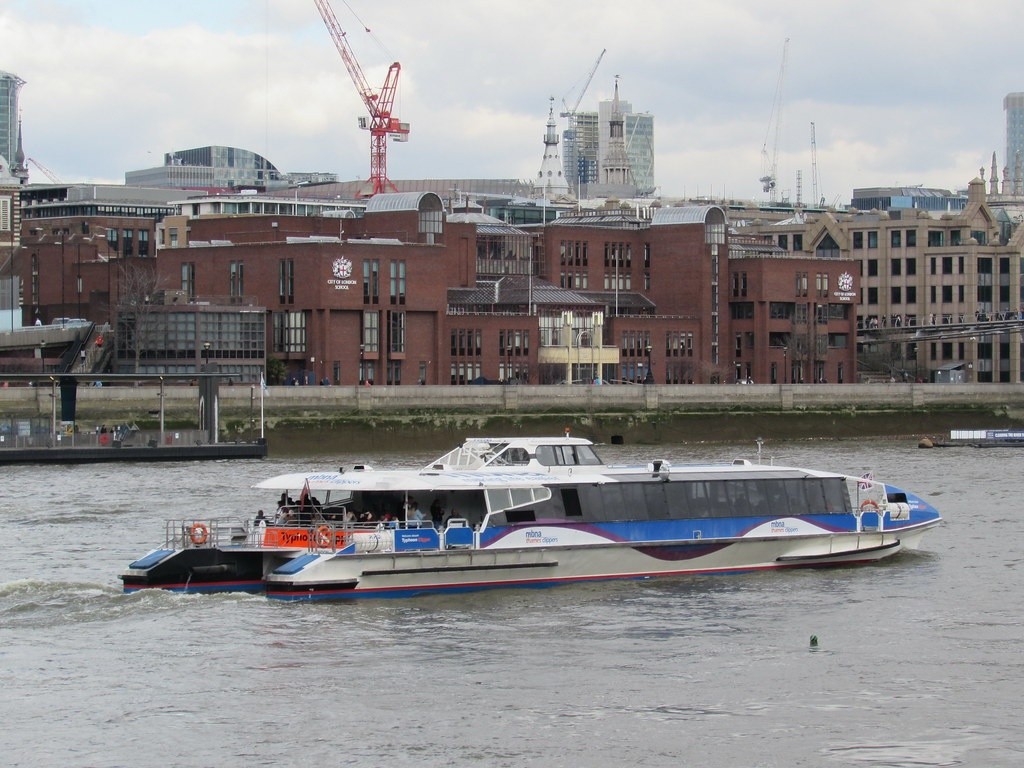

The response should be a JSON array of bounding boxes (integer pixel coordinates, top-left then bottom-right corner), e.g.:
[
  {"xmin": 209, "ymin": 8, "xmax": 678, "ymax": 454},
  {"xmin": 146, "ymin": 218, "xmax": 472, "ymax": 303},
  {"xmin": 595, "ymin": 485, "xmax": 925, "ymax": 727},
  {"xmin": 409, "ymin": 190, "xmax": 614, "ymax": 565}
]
[
  {"xmin": 858, "ymin": 473, "xmax": 874, "ymax": 490},
  {"xmin": 300, "ymin": 482, "xmax": 308, "ymax": 510},
  {"xmin": 262, "ymin": 378, "xmax": 270, "ymax": 396}
]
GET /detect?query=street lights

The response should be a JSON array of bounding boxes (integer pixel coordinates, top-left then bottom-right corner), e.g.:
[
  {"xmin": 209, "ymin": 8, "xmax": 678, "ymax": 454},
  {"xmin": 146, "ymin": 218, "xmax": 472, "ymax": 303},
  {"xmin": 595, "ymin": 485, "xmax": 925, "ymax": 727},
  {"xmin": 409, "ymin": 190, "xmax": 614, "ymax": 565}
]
[
  {"xmin": 914, "ymin": 345, "xmax": 919, "ymax": 383},
  {"xmin": 783, "ymin": 346, "xmax": 788, "ymax": 383},
  {"xmin": 645, "ymin": 343, "xmax": 655, "ymax": 384},
  {"xmin": 360, "ymin": 343, "xmax": 365, "ymax": 386},
  {"xmin": 204, "ymin": 340, "xmax": 211, "ymax": 366}
]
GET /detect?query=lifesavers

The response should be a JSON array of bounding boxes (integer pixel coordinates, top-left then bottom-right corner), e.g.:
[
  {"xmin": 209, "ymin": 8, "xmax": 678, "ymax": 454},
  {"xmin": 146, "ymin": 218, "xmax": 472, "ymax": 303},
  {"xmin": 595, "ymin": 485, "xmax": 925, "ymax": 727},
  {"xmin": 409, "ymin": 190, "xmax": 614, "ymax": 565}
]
[
  {"xmin": 860, "ymin": 499, "xmax": 878, "ymax": 511},
  {"xmin": 190, "ymin": 522, "xmax": 208, "ymax": 544},
  {"xmin": 315, "ymin": 526, "xmax": 332, "ymax": 548}
]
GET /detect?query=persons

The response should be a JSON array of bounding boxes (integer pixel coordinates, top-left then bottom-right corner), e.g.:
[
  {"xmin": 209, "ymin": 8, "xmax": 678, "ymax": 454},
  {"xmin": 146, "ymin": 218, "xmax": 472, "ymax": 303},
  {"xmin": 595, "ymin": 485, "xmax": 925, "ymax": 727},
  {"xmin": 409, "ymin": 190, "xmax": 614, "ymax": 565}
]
[
  {"xmin": 889, "ymin": 376, "xmax": 896, "ymax": 383},
  {"xmin": 693, "ymin": 376, "xmax": 838, "ymax": 384},
  {"xmin": 916, "ymin": 375, "xmax": 929, "ymax": 383},
  {"xmin": 35, "ymin": 318, "xmax": 42, "ymax": 326},
  {"xmin": 318, "ymin": 376, "xmax": 330, "ymax": 386},
  {"xmin": 857, "ymin": 307, "xmax": 1024, "ymax": 331},
  {"xmin": 250, "ymin": 492, "xmax": 467, "ymax": 527},
  {"xmin": 100, "ymin": 424, "xmax": 107, "ymax": 447},
  {"xmin": 901, "ymin": 370, "xmax": 909, "ymax": 383},
  {"xmin": 110, "ymin": 426, "xmax": 117, "ymax": 447},
  {"xmin": 291, "ymin": 377, "xmax": 300, "ymax": 386},
  {"xmin": 80, "ymin": 347, "xmax": 87, "ymax": 363}
]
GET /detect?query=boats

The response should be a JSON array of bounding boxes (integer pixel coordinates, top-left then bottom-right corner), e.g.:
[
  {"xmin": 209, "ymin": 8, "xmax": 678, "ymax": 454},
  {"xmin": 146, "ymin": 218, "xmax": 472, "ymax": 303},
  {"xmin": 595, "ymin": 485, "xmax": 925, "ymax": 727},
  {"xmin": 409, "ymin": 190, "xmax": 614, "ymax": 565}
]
[{"xmin": 116, "ymin": 429, "xmax": 942, "ymax": 603}]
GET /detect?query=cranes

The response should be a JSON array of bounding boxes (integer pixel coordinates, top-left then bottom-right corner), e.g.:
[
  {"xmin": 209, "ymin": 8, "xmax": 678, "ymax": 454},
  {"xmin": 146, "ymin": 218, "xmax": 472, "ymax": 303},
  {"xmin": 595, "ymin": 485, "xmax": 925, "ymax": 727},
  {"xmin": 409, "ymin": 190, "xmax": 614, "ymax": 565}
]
[
  {"xmin": 559, "ymin": 49, "xmax": 607, "ymax": 193},
  {"xmin": 314, "ymin": 0, "xmax": 410, "ymax": 196},
  {"xmin": 760, "ymin": 37, "xmax": 792, "ymax": 207}
]
